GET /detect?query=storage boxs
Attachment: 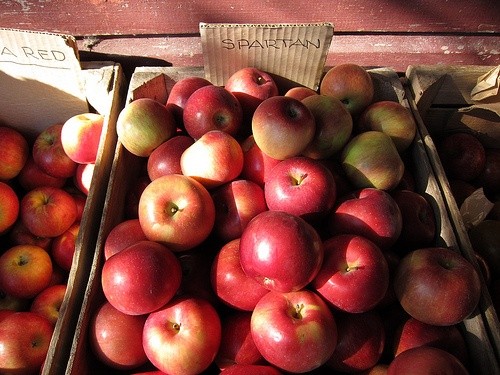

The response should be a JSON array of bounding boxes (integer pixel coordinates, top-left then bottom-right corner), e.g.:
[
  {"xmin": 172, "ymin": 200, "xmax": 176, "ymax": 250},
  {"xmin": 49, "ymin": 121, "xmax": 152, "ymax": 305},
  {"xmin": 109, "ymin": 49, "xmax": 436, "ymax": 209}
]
[{"xmin": 0, "ymin": 61, "xmax": 500, "ymax": 375}]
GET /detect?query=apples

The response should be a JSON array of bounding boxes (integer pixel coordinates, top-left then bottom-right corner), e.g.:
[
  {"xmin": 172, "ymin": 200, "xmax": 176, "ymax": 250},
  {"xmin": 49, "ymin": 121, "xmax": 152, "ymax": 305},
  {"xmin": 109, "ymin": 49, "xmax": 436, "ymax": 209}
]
[
  {"xmin": 440, "ymin": 131, "xmax": 500, "ymax": 318},
  {"xmin": 0, "ymin": 113, "xmax": 104, "ymax": 375},
  {"xmin": 90, "ymin": 63, "xmax": 480, "ymax": 375}
]
